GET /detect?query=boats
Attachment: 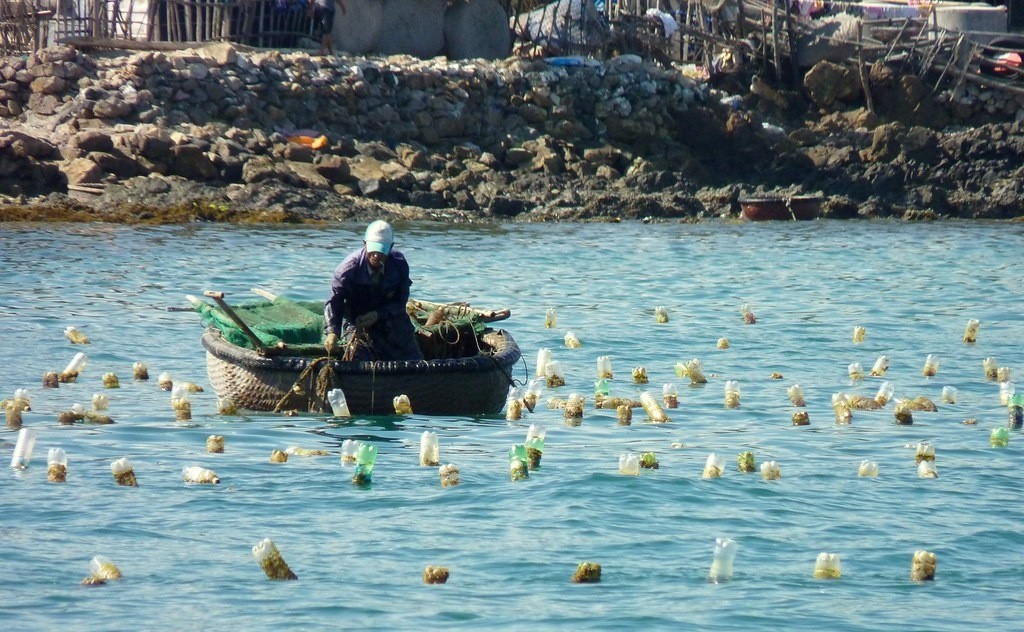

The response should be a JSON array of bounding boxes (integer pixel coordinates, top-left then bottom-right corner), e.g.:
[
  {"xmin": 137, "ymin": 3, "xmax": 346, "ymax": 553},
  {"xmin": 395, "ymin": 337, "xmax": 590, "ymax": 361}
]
[{"xmin": 201, "ymin": 297, "xmax": 522, "ymax": 417}]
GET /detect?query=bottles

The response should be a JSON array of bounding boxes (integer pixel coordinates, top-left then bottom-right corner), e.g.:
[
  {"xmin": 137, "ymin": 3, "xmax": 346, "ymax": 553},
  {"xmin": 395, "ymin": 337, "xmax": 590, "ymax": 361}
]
[
  {"xmin": 812, "ymin": 553, "xmax": 843, "ymax": 580},
  {"xmin": 1, "ymin": 304, "xmax": 1023, "ymax": 490},
  {"xmin": 571, "ymin": 561, "xmax": 602, "ymax": 583},
  {"xmin": 88, "ymin": 555, "xmax": 121, "ymax": 584},
  {"xmin": 910, "ymin": 549, "xmax": 936, "ymax": 582},
  {"xmin": 423, "ymin": 565, "xmax": 450, "ymax": 585},
  {"xmin": 707, "ymin": 537, "xmax": 737, "ymax": 583},
  {"xmin": 251, "ymin": 538, "xmax": 297, "ymax": 581}
]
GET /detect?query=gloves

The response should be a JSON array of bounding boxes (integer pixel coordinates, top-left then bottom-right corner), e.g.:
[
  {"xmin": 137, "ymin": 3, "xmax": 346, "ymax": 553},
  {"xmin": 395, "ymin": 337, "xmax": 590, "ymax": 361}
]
[
  {"xmin": 324, "ymin": 333, "xmax": 340, "ymax": 355},
  {"xmin": 356, "ymin": 310, "xmax": 377, "ymax": 331}
]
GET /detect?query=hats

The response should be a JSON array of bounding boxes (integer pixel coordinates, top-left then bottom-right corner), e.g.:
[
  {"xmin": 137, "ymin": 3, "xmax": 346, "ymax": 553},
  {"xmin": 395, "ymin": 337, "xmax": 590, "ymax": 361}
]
[{"xmin": 364, "ymin": 220, "xmax": 393, "ymax": 256}]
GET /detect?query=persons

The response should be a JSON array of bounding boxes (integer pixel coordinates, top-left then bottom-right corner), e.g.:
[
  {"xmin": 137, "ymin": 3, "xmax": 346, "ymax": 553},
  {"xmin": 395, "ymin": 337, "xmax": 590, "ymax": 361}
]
[{"xmin": 322, "ymin": 220, "xmax": 423, "ymax": 363}]
